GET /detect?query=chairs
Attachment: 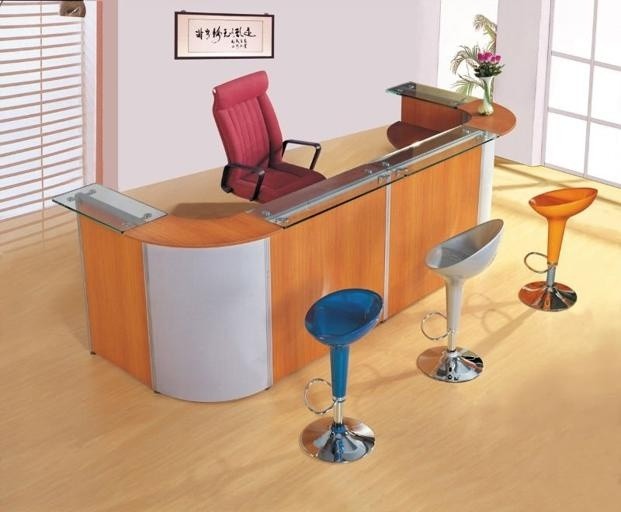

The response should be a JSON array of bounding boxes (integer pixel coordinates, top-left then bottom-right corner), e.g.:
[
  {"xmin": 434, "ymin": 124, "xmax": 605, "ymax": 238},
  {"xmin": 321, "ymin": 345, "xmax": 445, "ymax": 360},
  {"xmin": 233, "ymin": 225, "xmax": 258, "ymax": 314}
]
[
  {"xmin": 417, "ymin": 219, "xmax": 503, "ymax": 385},
  {"xmin": 518, "ymin": 187, "xmax": 598, "ymax": 312},
  {"xmin": 299, "ymin": 288, "xmax": 384, "ymax": 462},
  {"xmin": 211, "ymin": 70, "xmax": 326, "ymax": 203}
]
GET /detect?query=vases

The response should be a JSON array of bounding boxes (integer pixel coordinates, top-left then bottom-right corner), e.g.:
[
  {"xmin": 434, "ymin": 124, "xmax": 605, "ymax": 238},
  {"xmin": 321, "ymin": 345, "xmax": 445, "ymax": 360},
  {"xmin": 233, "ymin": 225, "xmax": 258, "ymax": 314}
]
[{"xmin": 477, "ymin": 75, "xmax": 495, "ymax": 115}]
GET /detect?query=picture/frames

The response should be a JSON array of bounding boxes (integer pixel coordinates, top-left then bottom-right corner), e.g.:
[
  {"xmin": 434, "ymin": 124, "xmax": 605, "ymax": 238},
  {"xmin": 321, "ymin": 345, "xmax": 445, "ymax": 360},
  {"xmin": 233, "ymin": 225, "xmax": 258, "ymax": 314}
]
[{"xmin": 174, "ymin": 10, "xmax": 274, "ymax": 59}]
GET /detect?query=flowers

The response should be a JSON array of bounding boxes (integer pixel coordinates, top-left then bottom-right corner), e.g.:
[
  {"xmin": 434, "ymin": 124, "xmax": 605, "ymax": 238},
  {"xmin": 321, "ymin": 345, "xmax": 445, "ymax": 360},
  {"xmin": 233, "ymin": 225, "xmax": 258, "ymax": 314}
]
[{"xmin": 473, "ymin": 52, "xmax": 505, "ymax": 76}]
